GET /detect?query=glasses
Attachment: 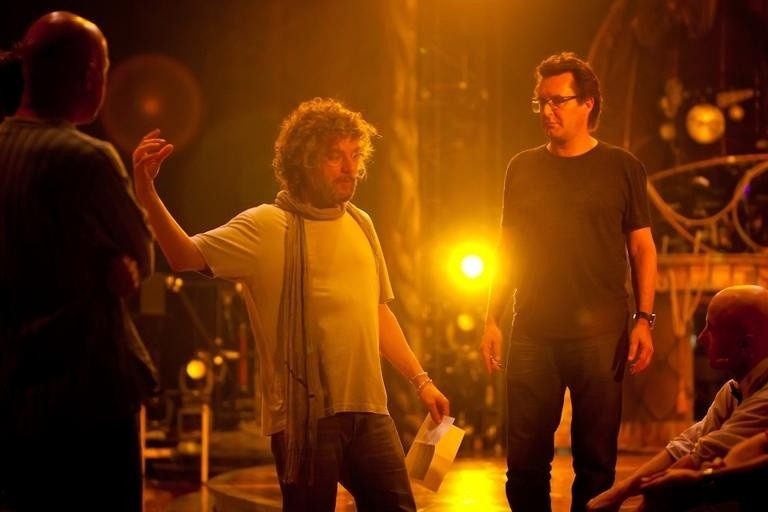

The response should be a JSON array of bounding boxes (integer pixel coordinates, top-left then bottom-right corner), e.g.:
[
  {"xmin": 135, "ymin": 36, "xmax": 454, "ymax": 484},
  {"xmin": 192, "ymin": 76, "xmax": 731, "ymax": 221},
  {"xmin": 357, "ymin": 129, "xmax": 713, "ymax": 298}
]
[{"xmin": 531, "ymin": 96, "xmax": 578, "ymax": 113}]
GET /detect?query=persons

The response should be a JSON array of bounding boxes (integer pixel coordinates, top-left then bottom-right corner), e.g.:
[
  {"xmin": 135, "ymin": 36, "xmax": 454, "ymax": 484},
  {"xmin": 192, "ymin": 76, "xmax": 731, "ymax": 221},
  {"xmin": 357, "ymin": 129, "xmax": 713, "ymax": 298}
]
[
  {"xmin": 0, "ymin": 10, "xmax": 154, "ymax": 512},
  {"xmin": 1, "ymin": 50, "xmax": 25, "ymax": 120},
  {"xmin": 638, "ymin": 427, "xmax": 768, "ymax": 511},
  {"xmin": 132, "ymin": 94, "xmax": 454, "ymax": 512},
  {"xmin": 482, "ymin": 51, "xmax": 659, "ymax": 512},
  {"xmin": 587, "ymin": 284, "xmax": 768, "ymax": 511}
]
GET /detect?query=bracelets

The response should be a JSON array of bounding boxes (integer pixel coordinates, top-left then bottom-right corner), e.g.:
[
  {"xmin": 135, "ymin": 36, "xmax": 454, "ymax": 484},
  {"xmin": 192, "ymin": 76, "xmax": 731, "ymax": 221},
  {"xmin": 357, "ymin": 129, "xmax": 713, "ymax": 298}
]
[
  {"xmin": 703, "ymin": 467, "xmax": 713, "ymax": 479},
  {"xmin": 416, "ymin": 377, "xmax": 430, "ymax": 392},
  {"xmin": 415, "ymin": 379, "xmax": 433, "ymax": 400},
  {"xmin": 635, "ymin": 312, "xmax": 657, "ymax": 329},
  {"xmin": 409, "ymin": 370, "xmax": 428, "ymax": 385}
]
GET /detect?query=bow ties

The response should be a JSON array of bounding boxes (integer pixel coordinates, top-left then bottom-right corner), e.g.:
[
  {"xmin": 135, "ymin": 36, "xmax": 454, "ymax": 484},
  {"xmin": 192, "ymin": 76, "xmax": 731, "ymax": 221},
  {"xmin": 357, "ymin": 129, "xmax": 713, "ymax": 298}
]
[{"xmin": 730, "ymin": 383, "xmax": 742, "ymax": 405}]
